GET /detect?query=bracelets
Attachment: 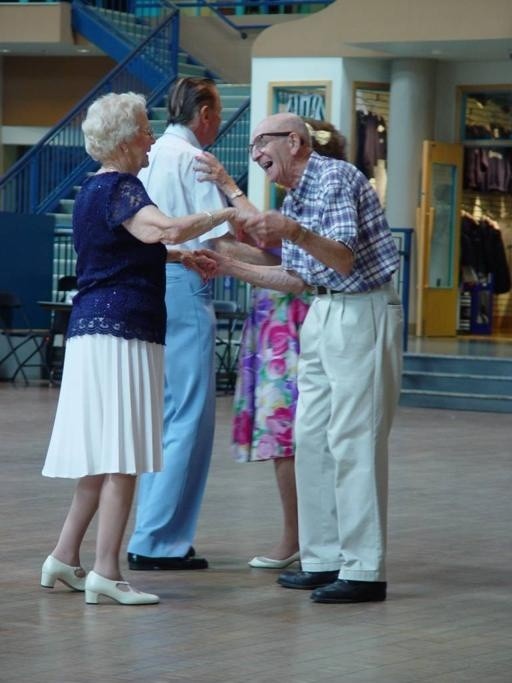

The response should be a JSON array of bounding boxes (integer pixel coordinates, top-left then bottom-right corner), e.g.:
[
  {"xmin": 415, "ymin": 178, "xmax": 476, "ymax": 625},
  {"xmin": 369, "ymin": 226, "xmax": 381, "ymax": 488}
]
[
  {"xmin": 200, "ymin": 210, "xmax": 213, "ymax": 231},
  {"xmin": 227, "ymin": 190, "xmax": 244, "ymax": 202}
]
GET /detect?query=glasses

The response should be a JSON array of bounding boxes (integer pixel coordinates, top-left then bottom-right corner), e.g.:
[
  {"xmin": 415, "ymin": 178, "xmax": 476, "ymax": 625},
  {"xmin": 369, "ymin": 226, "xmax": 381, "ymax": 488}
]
[{"xmin": 248, "ymin": 132, "xmax": 291, "ymax": 151}]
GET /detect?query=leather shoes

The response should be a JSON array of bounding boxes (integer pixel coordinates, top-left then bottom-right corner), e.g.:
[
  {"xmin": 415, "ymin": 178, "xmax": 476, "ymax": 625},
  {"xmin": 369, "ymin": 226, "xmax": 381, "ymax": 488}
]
[
  {"xmin": 248, "ymin": 550, "xmax": 301, "ymax": 567},
  {"xmin": 277, "ymin": 570, "xmax": 340, "ymax": 589},
  {"xmin": 310, "ymin": 581, "xmax": 388, "ymax": 603},
  {"xmin": 125, "ymin": 545, "xmax": 208, "ymax": 571}
]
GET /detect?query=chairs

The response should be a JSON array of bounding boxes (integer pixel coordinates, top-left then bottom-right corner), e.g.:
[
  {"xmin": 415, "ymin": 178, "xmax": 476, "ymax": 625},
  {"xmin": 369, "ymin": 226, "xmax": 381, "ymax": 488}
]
[
  {"xmin": 1, "ymin": 293, "xmax": 49, "ymax": 386},
  {"xmin": 213, "ymin": 300, "xmax": 242, "ymax": 394},
  {"xmin": 51, "ymin": 276, "xmax": 78, "ymax": 332}
]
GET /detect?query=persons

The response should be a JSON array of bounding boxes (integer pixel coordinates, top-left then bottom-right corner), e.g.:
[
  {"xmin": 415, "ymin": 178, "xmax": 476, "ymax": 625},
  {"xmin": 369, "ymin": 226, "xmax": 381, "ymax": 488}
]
[
  {"xmin": 194, "ymin": 114, "xmax": 407, "ymax": 601},
  {"xmin": 126, "ymin": 75, "xmax": 282, "ymax": 573},
  {"xmin": 37, "ymin": 93, "xmax": 260, "ymax": 606},
  {"xmin": 194, "ymin": 118, "xmax": 347, "ymax": 568}
]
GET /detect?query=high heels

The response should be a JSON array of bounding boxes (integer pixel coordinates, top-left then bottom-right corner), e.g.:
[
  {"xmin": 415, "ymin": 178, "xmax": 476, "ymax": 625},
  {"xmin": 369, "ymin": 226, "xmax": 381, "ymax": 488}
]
[
  {"xmin": 39, "ymin": 555, "xmax": 86, "ymax": 590},
  {"xmin": 84, "ymin": 570, "xmax": 160, "ymax": 605}
]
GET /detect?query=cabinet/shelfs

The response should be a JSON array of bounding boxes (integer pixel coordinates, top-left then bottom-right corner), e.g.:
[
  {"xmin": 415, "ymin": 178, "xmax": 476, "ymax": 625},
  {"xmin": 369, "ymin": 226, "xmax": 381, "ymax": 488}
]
[{"xmin": 460, "ymin": 281, "xmax": 496, "ymax": 336}]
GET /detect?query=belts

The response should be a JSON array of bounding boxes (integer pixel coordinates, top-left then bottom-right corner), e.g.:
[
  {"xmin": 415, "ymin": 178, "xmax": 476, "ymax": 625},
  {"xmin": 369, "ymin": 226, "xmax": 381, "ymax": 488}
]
[{"xmin": 313, "ymin": 284, "xmax": 344, "ymax": 295}]
